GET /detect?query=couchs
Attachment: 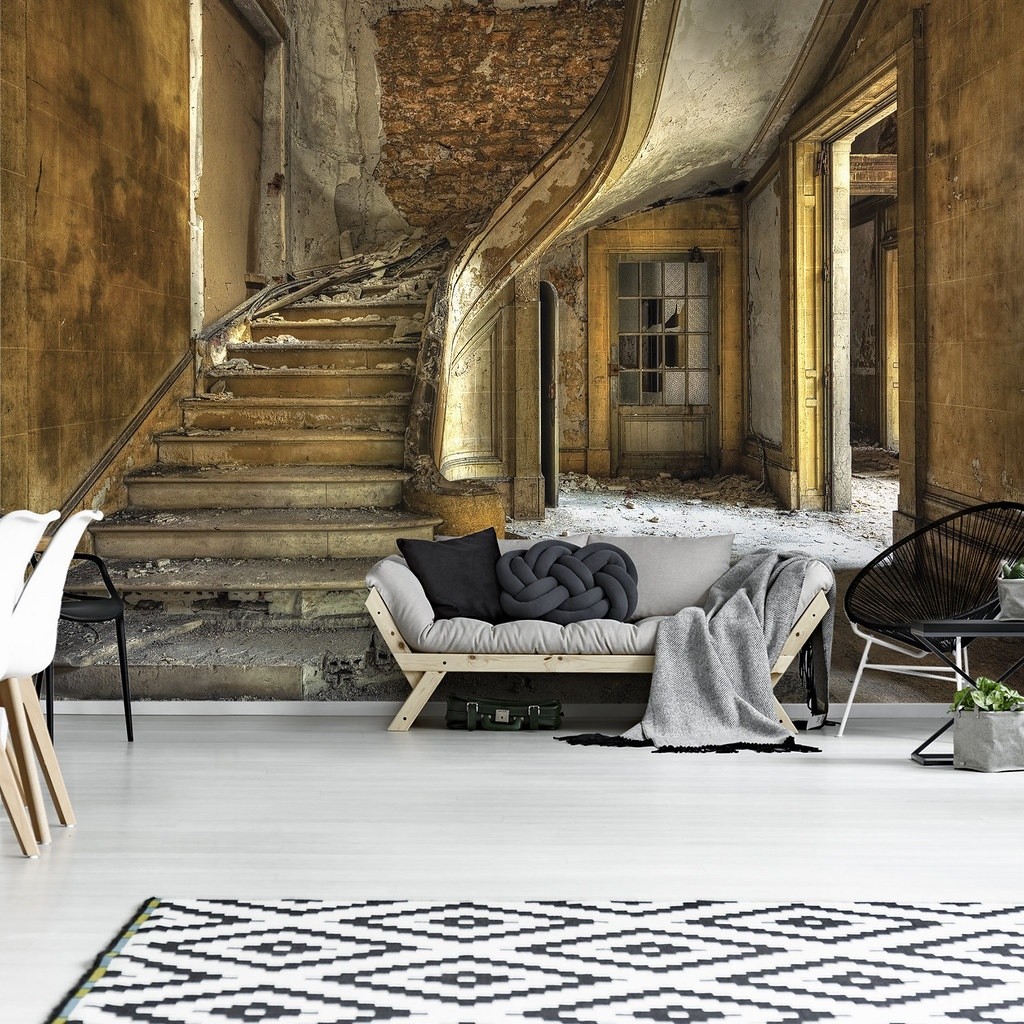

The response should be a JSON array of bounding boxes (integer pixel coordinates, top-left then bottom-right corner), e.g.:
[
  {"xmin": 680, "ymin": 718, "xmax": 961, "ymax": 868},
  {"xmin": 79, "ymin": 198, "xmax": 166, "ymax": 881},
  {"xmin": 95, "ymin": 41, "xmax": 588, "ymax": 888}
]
[{"xmin": 361, "ymin": 548, "xmax": 839, "ymax": 753}]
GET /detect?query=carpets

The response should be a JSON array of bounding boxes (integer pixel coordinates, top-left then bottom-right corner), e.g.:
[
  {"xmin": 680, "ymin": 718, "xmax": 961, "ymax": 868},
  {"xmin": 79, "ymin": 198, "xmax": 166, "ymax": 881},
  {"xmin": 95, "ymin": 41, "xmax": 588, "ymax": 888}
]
[{"xmin": 47, "ymin": 893, "xmax": 1024, "ymax": 1024}]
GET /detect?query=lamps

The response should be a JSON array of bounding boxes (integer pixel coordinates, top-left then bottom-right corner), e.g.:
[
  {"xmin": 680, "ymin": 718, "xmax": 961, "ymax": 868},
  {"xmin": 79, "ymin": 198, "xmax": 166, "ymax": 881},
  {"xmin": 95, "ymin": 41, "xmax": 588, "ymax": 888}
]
[{"xmin": 688, "ymin": 245, "xmax": 705, "ymax": 263}]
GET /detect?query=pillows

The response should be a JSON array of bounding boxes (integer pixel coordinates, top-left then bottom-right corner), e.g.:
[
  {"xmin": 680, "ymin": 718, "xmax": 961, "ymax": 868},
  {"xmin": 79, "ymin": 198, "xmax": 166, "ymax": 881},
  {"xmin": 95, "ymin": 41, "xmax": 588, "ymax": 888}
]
[
  {"xmin": 393, "ymin": 527, "xmax": 504, "ymax": 620},
  {"xmin": 434, "ymin": 535, "xmax": 589, "ymax": 555},
  {"xmin": 589, "ymin": 534, "xmax": 736, "ymax": 618}
]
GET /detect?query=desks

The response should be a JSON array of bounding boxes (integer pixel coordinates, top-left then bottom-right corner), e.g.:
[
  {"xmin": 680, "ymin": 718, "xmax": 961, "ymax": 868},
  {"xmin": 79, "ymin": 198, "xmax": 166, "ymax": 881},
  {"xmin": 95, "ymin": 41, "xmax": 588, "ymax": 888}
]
[{"xmin": 907, "ymin": 621, "xmax": 1024, "ymax": 765}]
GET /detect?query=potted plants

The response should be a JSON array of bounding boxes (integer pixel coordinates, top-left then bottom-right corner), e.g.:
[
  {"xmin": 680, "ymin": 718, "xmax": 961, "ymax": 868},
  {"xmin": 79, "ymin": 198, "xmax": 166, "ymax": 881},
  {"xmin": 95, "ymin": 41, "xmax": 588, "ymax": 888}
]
[
  {"xmin": 943, "ymin": 677, "xmax": 1024, "ymax": 773},
  {"xmin": 997, "ymin": 562, "xmax": 1024, "ymax": 620}
]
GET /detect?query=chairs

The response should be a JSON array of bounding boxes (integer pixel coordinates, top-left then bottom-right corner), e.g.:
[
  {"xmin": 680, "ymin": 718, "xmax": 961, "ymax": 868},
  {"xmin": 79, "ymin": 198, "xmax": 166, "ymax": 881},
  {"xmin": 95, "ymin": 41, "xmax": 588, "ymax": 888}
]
[
  {"xmin": 0, "ymin": 507, "xmax": 61, "ymax": 859},
  {"xmin": 835, "ymin": 502, "xmax": 1024, "ymax": 739},
  {"xmin": 13, "ymin": 509, "xmax": 103, "ymax": 833}
]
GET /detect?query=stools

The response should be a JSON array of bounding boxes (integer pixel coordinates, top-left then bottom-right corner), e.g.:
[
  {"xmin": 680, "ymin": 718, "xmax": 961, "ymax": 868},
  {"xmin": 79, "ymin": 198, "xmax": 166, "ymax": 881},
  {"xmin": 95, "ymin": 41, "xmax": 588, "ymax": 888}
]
[{"xmin": 27, "ymin": 552, "xmax": 134, "ymax": 746}]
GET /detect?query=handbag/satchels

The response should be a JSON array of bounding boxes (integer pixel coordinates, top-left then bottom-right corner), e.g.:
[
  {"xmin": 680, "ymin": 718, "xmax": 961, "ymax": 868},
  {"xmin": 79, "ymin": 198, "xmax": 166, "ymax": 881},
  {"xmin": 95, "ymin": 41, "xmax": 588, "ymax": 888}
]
[
  {"xmin": 994, "ymin": 559, "xmax": 1024, "ymax": 621},
  {"xmin": 954, "ymin": 687, "xmax": 1024, "ymax": 773},
  {"xmin": 442, "ymin": 692, "xmax": 564, "ymax": 731}
]
